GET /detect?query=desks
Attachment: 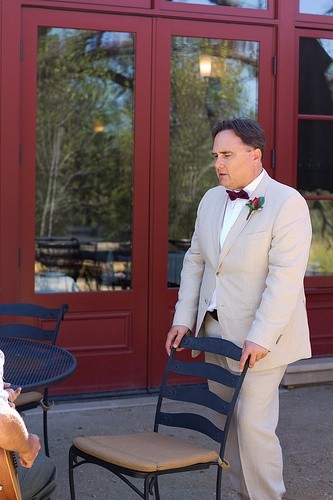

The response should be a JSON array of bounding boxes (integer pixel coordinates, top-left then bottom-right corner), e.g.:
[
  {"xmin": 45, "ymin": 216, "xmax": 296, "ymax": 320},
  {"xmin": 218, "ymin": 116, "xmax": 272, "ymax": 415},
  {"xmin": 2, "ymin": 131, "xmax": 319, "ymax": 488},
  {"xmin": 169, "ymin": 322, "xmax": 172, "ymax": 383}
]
[
  {"xmin": 0, "ymin": 336, "xmax": 77, "ymax": 413},
  {"xmin": 167, "ymin": 252, "xmax": 187, "ymax": 285},
  {"xmin": 79, "ymin": 250, "xmax": 131, "ymax": 290}
]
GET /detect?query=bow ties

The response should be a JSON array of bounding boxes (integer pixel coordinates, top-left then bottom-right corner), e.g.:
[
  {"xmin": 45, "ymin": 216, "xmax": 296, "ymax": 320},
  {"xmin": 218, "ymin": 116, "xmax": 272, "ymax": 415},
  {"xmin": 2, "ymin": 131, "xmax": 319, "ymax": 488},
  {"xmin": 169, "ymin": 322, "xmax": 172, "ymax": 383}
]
[{"xmin": 226, "ymin": 189, "xmax": 249, "ymax": 201}]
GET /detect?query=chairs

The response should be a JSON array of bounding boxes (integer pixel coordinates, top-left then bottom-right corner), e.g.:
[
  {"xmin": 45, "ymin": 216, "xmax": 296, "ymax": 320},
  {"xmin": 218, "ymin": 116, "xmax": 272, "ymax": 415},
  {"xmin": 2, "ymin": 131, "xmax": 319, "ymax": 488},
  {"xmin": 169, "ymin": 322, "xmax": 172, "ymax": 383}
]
[
  {"xmin": 69, "ymin": 335, "xmax": 250, "ymax": 500},
  {"xmin": 0, "ymin": 304, "xmax": 68, "ymax": 456},
  {"xmin": 34, "ymin": 237, "xmax": 131, "ymax": 290}
]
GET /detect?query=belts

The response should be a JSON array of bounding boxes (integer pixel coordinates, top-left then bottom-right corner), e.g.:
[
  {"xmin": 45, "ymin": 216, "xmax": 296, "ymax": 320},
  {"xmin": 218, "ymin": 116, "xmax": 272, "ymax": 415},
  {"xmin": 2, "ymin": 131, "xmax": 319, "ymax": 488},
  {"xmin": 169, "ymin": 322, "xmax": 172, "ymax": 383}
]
[{"xmin": 207, "ymin": 309, "xmax": 219, "ymax": 321}]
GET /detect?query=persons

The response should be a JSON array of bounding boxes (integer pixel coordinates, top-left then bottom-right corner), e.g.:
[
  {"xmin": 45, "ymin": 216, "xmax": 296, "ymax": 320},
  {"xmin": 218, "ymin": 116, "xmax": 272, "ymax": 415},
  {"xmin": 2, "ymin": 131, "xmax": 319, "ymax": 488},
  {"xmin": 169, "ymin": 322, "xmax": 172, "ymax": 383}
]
[
  {"xmin": 34, "ymin": 259, "xmax": 131, "ymax": 291},
  {"xmin": 0, "ymin": 350, "xmax": 62, "ymax": 500},
  {"xmin": 165, "ymin": 118, "xmax": 312, "ymax": 500}
]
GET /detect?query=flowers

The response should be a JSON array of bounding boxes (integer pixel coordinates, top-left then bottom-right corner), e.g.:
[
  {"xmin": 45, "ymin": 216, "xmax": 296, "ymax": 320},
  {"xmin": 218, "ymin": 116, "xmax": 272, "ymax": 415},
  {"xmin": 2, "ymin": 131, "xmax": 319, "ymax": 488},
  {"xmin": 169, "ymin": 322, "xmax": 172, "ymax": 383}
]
[{"xmin": 246, "ymin": 196, "xmax": 265, "ymax": 221}]
